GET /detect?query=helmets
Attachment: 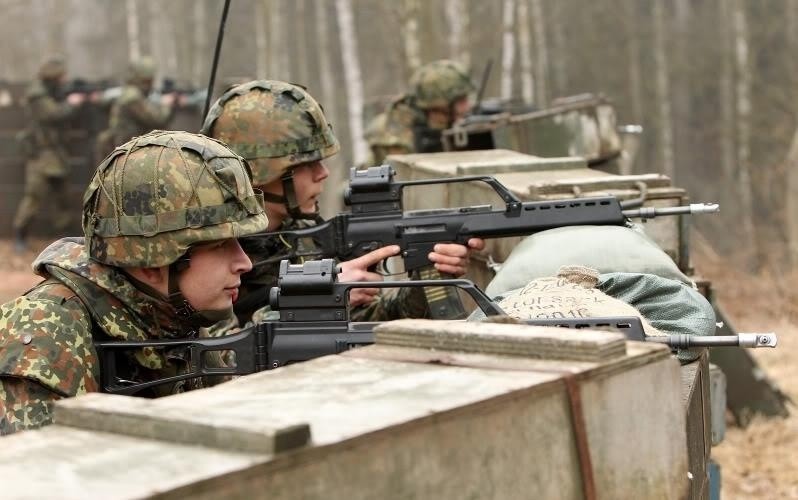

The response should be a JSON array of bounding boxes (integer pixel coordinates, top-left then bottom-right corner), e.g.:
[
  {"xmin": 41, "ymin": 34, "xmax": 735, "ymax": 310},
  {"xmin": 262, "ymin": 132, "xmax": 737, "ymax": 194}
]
[
  {"xmin": 198, "ymin": 78, "xmax": 341, "ymax": 190},
  {"xmin": 80, "ymin": 130, "xmax": 269, "ymax": 268},
  {"xmin": 128, "ymin": 54, "xmax": 158, "ymax": 82},
  {"xmin": 407, "ymin": 58, "xmax": 475, "ymax": 110},
  {"xmin": 36, "ymin": 51, "xmax": 69, "ymax": 80}
]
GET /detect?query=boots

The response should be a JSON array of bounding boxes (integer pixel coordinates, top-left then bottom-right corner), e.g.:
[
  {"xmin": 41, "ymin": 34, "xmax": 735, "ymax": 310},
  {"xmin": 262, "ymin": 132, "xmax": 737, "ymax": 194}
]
[{"xmin": 13, "ymin": 226, "xmax": 28, "ymax": 256}]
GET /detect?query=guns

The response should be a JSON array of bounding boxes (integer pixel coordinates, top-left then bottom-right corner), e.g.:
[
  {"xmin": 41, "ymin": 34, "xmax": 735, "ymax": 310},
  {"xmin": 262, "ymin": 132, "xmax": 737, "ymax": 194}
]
[
  {"xmin": 94, "ymin": 259, "xmax": 778, "ymax": 394},
  {"xmin": 249, "ymin": 163, "xmax": 721, "ymax": 319}
]
